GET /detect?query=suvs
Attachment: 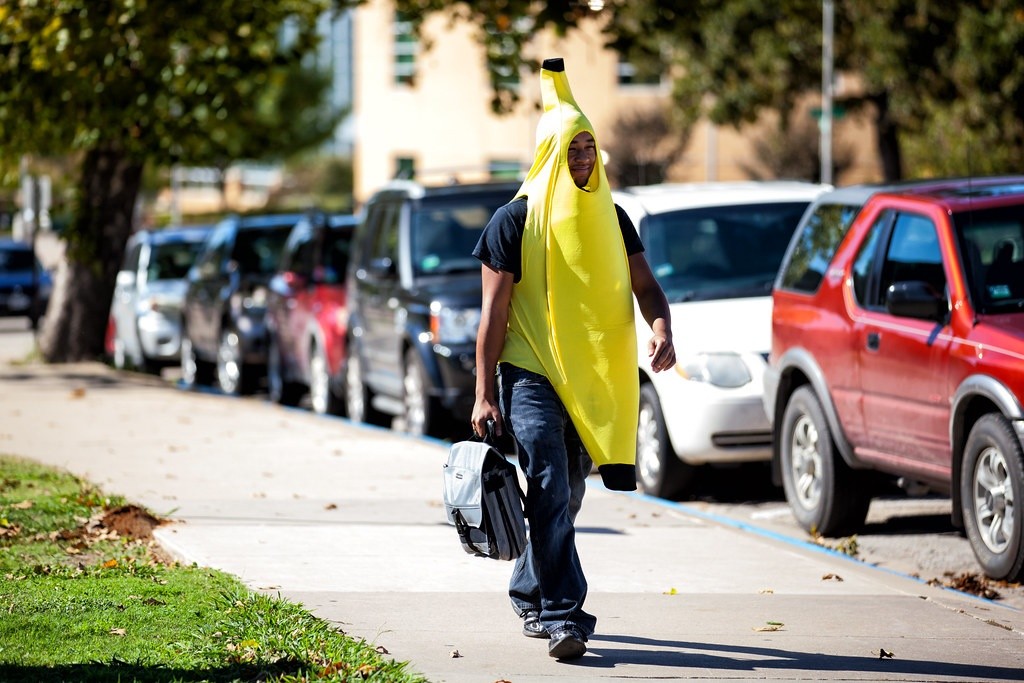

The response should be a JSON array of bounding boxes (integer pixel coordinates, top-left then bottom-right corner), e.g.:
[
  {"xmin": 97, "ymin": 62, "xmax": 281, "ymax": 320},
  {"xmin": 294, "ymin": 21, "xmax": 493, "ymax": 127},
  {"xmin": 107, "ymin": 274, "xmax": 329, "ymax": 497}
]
[
  {"xmin": 764, "ymin": 178, "xmax": 1023, "ymax": 583},
  {"xmin": 342, "ymin": 178, "xmax": 523, "ymax": 435}
]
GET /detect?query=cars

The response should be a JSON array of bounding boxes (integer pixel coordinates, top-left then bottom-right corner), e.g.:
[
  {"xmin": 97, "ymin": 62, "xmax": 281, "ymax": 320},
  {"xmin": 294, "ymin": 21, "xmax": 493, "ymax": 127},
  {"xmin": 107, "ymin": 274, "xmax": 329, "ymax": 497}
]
[
  {"xmin": 175, "ymin": 214, "xmax": 303, "ymax": 396},
  {"xmin": 595, "ymin": 181, "xmax": 836, "ymax": 501},
  {"xmin": 268, "ymin": 216, "xmax": 365, "ymax": 414},
  {"xmin": 110, "ymin": 230, "xmax": 209, "ymax": 376},
  {"xmin": 0, "ymin": 240, "xmax": 54, "ymax": 326}
]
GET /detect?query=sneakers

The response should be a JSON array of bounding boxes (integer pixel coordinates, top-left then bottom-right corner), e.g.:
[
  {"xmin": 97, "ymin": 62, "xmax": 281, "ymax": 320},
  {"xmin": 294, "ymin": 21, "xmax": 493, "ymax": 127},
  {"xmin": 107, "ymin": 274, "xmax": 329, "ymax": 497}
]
[
  {"xmin": 548, "ymin": 611, "xmax": 587, "ymax": 659},
  {"xmin": 510, "ymin": 598, "xmax": 550, "ymax": 638}
]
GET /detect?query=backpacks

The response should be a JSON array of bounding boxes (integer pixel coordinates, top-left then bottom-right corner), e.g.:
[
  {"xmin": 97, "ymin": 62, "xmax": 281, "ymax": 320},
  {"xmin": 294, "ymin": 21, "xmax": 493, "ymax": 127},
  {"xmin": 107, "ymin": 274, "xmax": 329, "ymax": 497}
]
[{"xmin": 442, "ymin": 421, "xmax": 528, "ymax": 561}]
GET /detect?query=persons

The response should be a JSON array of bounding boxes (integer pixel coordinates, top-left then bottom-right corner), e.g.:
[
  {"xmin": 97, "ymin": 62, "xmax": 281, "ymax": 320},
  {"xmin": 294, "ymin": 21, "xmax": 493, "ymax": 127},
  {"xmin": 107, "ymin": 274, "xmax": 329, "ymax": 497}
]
[{"xmin": 470, "ymin": 103, "xmax": 677, "ymax": 661}]
